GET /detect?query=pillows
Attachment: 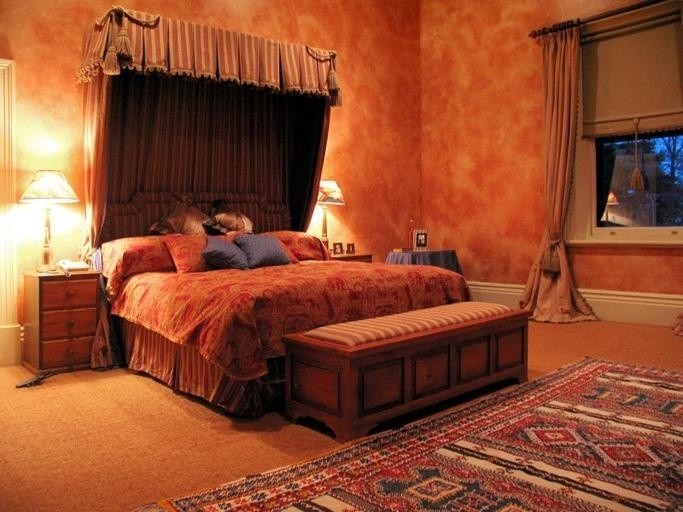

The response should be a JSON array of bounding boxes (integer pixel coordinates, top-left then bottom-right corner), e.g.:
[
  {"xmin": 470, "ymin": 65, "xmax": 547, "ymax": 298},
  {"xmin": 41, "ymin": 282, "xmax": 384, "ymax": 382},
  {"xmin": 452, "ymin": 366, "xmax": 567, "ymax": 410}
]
[{"xmin": 151, "ymin": 195, "xmax": 300, "ymax": 275}]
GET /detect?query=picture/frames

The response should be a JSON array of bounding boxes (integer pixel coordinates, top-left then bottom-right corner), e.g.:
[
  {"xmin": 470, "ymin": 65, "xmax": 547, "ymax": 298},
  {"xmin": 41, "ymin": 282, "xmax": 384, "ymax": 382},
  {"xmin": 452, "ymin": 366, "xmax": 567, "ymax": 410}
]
[{"xmin": 412, "ymin": 229, "xmax": 430, "ymax": 252}]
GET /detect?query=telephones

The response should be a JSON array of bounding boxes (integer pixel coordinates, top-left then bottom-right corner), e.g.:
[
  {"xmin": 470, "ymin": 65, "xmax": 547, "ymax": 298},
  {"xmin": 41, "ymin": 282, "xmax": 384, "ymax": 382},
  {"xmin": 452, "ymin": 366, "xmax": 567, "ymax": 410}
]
[{"xmin": 57, "ymin": 260, "xmax": 91, "ymax": 271}]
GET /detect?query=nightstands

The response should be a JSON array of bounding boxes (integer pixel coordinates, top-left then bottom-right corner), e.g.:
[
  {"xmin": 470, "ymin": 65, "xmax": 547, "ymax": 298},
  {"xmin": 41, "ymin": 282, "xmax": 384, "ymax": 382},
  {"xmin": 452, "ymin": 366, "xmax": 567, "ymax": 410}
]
[
  {"xmin": 333, "ymin": 254, "xmax": 374, "ymax": 265},
  {"xmin": 20, "ymin": 268, "xmax": 103, "ymax": 379}
]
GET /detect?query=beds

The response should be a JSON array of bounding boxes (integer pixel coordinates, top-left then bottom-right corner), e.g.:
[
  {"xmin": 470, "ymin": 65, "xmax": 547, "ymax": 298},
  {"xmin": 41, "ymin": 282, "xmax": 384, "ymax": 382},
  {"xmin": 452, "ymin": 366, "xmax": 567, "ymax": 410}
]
[{"xmin": 95, "ymin": 193, "xmax": 471, "ymax": 420}]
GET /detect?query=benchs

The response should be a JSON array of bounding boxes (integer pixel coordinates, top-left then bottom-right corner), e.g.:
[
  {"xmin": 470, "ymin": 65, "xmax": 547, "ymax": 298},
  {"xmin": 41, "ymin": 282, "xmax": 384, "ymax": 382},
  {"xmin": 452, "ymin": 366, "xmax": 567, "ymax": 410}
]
[{"xmin": 281, "ymin": 298, "xmax": 534, "ymax": 443}]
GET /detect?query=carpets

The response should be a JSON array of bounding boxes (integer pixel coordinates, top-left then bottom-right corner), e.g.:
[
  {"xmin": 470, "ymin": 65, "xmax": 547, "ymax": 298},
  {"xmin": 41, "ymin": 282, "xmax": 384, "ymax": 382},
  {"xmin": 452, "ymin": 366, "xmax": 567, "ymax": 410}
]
[{"xmin": 114, "ymin": 353, "xmax": 683, "ymax": 510}]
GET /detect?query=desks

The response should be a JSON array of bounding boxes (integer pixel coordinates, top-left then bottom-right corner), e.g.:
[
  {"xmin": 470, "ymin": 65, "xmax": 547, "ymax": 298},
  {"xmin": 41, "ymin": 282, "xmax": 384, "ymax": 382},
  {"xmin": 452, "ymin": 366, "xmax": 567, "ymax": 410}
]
[{"xmin": 387, "ymin": 250, "xmax": 460, "ymax": 274}]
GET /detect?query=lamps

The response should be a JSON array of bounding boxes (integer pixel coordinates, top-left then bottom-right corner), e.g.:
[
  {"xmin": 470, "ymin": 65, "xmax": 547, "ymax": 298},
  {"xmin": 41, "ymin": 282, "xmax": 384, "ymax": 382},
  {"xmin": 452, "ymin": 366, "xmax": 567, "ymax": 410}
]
[
  {"xmin": 16, "ymin": 169, "xmax": 80, "ymax": 274},
  {"xmin": 315, "ymin": 180, "xmax": 346, "ymax": 251}
]
